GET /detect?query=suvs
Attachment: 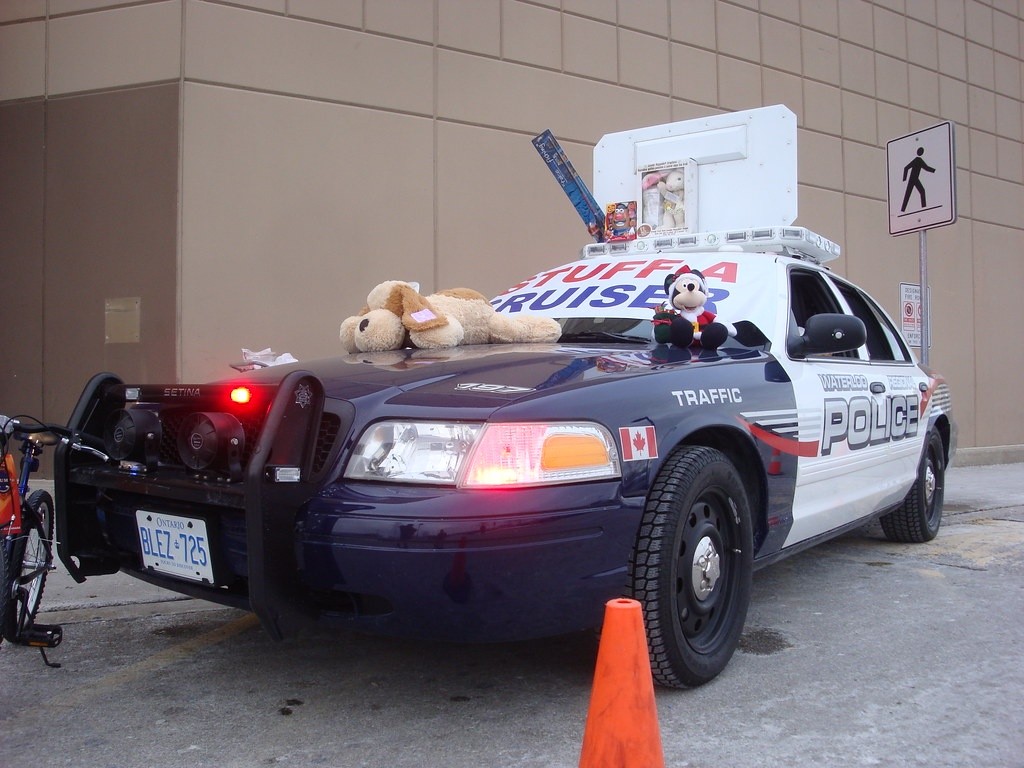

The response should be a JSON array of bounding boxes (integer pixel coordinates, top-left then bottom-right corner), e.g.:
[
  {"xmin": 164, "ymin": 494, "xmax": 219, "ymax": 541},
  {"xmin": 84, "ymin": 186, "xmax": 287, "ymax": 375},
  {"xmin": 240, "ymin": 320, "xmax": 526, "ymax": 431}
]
[{"xmin": 50, "ymin": 106, "xmax": 956, "ymax": 688}]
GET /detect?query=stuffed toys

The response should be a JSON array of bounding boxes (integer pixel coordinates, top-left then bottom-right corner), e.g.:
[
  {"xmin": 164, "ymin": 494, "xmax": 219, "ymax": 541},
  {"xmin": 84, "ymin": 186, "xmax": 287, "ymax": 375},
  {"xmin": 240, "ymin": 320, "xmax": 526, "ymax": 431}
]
[
  {"xmin": 651, "ymin": 265, "xmax": 739, "ymax": 347},
  {"xmin": 657, "ymin": 168, "xmax": 685, "ymax": 228},
  {"xmin": 339, "ymin": 279, "xmax": 562, "ymax": 352}
]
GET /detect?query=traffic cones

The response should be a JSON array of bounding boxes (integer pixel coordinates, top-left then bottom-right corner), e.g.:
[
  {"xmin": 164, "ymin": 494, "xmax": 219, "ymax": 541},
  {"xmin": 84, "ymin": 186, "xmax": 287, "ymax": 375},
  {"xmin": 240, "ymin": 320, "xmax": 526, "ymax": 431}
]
[{"xmin": 580, "ymin": 598, "xmax": 670, "ymax": 768}]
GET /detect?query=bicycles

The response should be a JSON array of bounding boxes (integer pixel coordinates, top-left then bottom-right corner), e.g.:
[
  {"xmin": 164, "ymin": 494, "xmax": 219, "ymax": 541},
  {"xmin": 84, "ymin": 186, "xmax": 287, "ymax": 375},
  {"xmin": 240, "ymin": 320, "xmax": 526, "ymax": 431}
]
[{"xmin": 0, "ymin": 415, "xmax": 112, "ymax": 672}]
